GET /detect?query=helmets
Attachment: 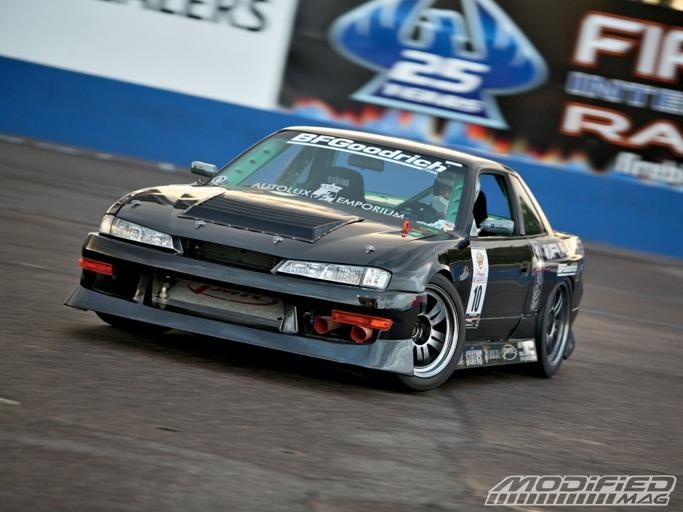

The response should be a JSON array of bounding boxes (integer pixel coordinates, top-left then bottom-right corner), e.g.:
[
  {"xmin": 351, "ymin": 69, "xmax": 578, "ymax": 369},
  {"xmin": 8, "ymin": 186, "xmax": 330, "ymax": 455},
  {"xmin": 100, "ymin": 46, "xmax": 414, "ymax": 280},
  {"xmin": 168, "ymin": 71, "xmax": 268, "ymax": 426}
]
[{"xmin": 430, "ymin": 169, "xmax": 480, "ymax": 215}]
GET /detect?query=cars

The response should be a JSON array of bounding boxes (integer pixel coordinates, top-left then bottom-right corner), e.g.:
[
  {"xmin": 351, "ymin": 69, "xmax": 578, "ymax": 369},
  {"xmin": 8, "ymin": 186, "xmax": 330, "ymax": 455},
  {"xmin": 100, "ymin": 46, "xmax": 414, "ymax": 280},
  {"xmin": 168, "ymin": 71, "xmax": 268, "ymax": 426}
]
[{"xmin": 68, "ymin": 121, "xmax": 587, "ymax": 383}]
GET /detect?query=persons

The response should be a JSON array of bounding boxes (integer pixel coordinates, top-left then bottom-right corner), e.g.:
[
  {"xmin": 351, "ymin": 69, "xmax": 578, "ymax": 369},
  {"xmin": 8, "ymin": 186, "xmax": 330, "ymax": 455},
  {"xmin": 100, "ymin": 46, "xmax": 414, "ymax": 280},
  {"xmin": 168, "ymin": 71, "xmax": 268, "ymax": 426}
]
[{"xmin": 397, "ymin": 169, "xmax": 480, "ymax": 237}]
